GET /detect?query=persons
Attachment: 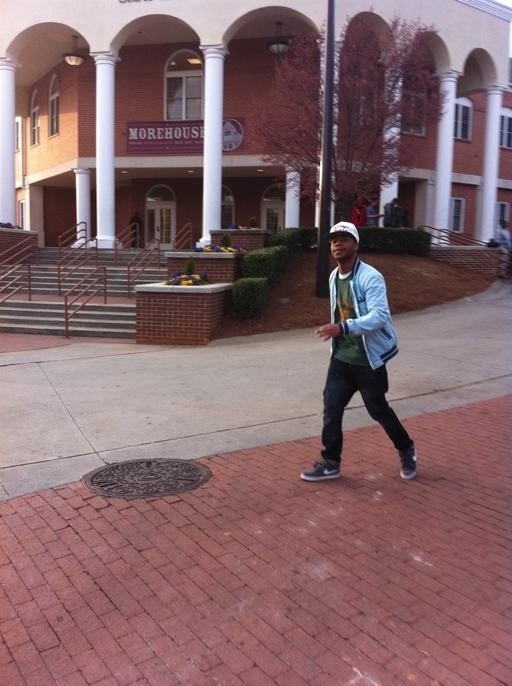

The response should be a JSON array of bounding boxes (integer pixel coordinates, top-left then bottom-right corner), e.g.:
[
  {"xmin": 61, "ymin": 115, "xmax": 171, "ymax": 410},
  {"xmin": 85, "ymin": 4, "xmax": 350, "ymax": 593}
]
[
  {"xmin": 352, "ymin": 197, "xmax": 405, "ymax": 228},
  {"xmin": 129, "ymin": 211, "xmax": 143, "ymax": 250},
  {"xmin": 493, "ymin": 220, "xmax": 511, "ymax": 279},
  {"xmin": 297, "ymin": 219, "xmax": 418, "ymax": 483}
]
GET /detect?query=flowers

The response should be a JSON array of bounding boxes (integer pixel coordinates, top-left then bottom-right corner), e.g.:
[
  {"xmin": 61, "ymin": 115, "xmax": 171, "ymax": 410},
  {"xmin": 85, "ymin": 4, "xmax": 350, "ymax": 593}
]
[
  {"xmin": 192, "ymin": 243, "xmax": 246, "ymax": 253},
  {"xmin": 166, "ymin": 271, "xmax": 210, "ymax": 285}
]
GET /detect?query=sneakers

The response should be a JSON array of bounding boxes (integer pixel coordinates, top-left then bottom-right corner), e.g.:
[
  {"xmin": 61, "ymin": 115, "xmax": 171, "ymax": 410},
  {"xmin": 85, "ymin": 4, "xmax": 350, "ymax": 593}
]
[
  {"xmin": 399, "ymin": 446, "xmax": 418, "ymax": 480},
  {"xmin": 300, "ymin": 460, "xmax": 341, "ymax": 481}
]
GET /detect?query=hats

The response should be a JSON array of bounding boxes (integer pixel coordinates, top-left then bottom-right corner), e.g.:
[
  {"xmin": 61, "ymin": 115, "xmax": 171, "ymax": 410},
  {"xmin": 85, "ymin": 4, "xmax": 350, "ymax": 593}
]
[{"xmin": 328, "ymin": 220, "xmax": 361, "ymax": 245}]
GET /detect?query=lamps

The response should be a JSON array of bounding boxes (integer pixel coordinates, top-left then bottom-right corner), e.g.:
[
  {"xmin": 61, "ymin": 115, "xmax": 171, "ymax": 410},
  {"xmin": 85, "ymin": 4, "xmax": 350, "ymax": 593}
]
[
  {"xmin": 63, "ymin": 34, "xmax": 86, "ymax": 66},
  {"xmin": 266, "ymin": 21, "xmax": 289, "ymax": 56}
]
